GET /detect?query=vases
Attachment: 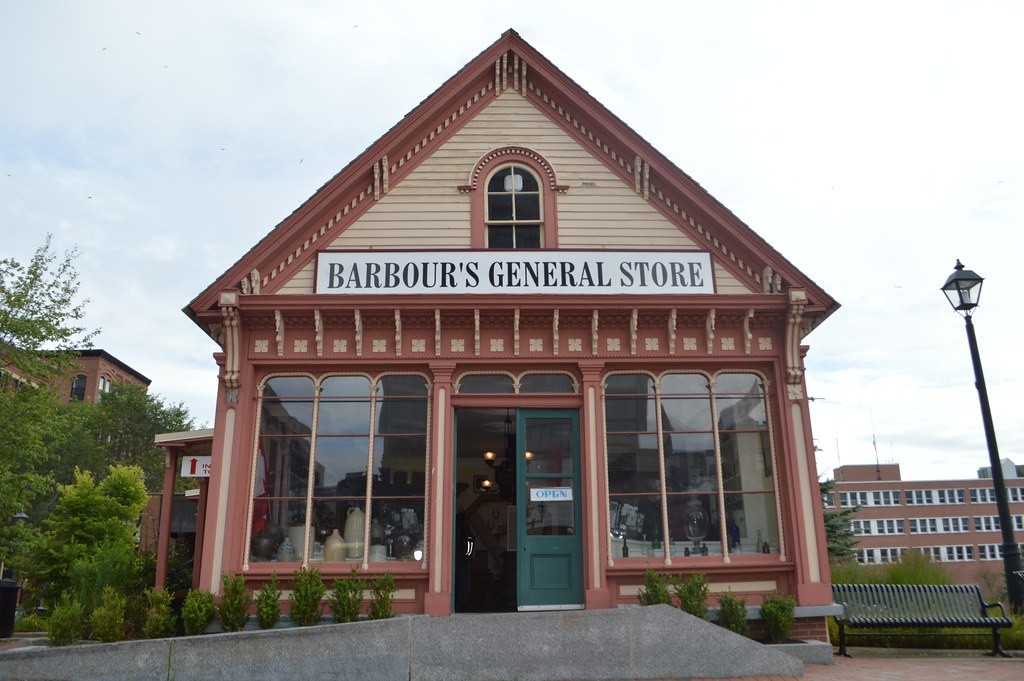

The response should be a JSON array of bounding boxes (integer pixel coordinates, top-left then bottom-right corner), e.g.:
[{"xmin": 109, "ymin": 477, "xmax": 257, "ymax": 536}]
[
  {"xmin": 370, "ymin": 537, "xmax": 387, "ymax": 561},
  {"xmin": 344, "ymin": 508, "xmax": 366, "ymax": 558},
  {"xmin": 324, "ymin": 529, "xmax": 349, "ymax": 561},
  {"xmin": 278, "ymin": 537, "xmax": 296, "ymax": 563},
  {"xmin": 371, "ymin": 517, "xmax": 386, "ymax": 545}
]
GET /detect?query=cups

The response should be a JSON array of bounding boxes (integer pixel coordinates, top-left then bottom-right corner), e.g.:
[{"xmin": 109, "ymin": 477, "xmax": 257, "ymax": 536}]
[
  {"xmin": 288, "ymin": 527, "xmax": 315, "ymax": 559},
  {"xmin": 370, "ymin": 545, "xmax": 387, "ymax": 561}
]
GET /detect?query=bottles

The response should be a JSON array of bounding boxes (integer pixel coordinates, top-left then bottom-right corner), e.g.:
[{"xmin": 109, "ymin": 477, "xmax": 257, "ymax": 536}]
[
  {"xmin": 763, "ymin": 542, "xmax": 770, "ymax": 554},
  {"xmin": 729, "ymin": 519, "xmax": 739, "ymax": 552},
  {"xmin": 324, "ymin": 529, "xmax": 346, "ymax": 562},
  {"xmin": 641, "ymin": 534, "xmax": 649, "ymax": 554},
  {"xmin": 651, "ymin": 530, "xmax": 661, "ymax": 549},
  {"xmin": 622, "ymin": 538, "xmax": 628, "ymax": 557},
  {"xmin": 373, "ymin": 537, "xmax": 383, "ymax": 545},
  {"xmin": 682, "ymin": 500, "xmax": 709, "ymax": 540},
  {"xmin": 701, "ymin": 543, "xmax": 708, "ymax": 556},
  {"xmin": 277, "ymin": 537, "xmax": 295, "ymax": 562},
  {"xmin": 395, "ymin": 529, "xmax": 417, "ymax": 560},
  {"xmin": 344, "ymin": 507, "xmax": 365, "ymax": 557},
  {"xmin": 684, "ymin": 548, "xmax": 690, "ymax": 556},
  {"xmin": 371, "ymin": 518, "xmax": 385, "ymax": 541}
]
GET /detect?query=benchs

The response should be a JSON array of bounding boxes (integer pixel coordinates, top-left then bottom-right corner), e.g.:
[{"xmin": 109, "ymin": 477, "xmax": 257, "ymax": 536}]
[{"xmin": 831, "ymin": 582, "xmax": 1016, "ymax": 659}]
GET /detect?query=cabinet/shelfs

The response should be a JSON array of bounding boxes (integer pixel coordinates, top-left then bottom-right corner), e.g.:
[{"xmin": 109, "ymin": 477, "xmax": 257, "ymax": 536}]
[{"xmin": 704, "ymin": 399, "xmax": 769, "ymax": 543}]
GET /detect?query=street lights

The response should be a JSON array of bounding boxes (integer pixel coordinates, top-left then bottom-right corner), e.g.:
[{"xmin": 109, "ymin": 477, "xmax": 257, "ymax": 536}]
[{"xmin": 939, "ymin": 258, "xmax": 1024, "ymax": 616}]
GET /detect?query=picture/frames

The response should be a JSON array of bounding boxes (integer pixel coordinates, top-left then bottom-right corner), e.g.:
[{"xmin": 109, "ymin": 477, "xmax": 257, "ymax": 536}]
[{"xmin": 472, "ymin": 474, "xmax": 487, "ymax": 493}]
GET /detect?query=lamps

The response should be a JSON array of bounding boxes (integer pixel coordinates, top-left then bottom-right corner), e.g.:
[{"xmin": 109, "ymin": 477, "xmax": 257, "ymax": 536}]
[{"xmin": 483, "ymin": 407, "xmax": 535, "ymax": 500}]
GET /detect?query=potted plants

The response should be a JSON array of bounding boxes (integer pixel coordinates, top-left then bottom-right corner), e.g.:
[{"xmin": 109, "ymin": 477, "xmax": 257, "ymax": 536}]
[{"xmin": 647, "ymin": 538, "xmax": 665, "ymax": 558}]
